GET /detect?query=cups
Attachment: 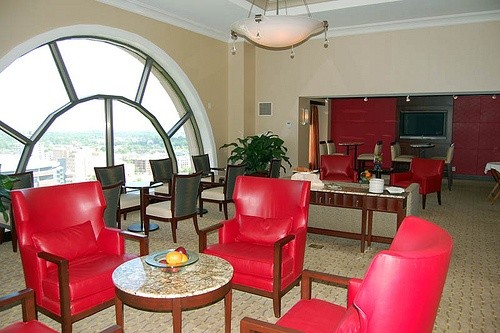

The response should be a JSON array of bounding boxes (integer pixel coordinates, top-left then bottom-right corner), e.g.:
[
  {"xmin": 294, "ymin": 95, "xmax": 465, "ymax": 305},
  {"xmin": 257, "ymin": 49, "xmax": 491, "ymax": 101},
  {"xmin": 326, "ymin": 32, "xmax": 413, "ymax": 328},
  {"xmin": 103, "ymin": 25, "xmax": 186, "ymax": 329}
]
[{"xmin": 369, "ymin": 178, "xmax": 384, "ymax": 193}]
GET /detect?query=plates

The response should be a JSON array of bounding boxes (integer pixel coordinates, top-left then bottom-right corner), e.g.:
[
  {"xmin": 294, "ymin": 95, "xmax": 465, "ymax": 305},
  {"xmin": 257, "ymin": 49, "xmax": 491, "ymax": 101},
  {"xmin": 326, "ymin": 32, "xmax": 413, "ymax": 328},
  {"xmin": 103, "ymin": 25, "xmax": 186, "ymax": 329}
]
[{"xmin": 386, "ymin": 187, "xmax": 404, "ymax": 193}]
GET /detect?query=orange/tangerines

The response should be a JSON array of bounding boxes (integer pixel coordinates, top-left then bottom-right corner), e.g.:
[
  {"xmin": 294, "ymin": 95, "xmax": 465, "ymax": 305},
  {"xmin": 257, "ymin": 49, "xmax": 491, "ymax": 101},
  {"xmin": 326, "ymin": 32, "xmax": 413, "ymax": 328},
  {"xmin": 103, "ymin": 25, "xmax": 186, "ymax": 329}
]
[{"xmin": 166, "ymin": 252, "xmax": 188, "ymax": 264}]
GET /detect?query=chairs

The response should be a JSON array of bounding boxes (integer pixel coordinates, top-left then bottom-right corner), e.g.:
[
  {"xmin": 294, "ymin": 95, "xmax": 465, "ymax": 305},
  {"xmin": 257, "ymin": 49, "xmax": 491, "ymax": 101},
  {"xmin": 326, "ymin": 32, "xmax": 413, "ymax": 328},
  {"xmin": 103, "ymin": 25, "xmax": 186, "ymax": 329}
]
[{"xmin": 0, "ymin": 140, "xmax": 455, "ymax": 333}]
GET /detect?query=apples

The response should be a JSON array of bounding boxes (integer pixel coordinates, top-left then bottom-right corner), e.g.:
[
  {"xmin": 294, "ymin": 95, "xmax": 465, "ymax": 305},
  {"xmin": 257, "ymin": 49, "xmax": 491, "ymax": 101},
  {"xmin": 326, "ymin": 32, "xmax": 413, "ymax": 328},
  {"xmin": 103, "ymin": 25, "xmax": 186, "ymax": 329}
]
[{"xmin": 175, "ymin": 247, "xmax": 186, "ymax": 254}]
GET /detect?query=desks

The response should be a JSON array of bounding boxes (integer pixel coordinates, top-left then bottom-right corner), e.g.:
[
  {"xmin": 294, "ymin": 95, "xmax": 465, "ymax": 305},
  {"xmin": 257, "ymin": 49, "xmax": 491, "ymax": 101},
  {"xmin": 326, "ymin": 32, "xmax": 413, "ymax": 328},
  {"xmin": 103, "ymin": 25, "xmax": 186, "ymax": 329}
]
[
  {"xmin": 484, "ymin": 162, "xmax": 500, "ymax": 207},
  {"xmin": 410, "ymin": 143, "xmax": 437, "ymax": 159},
  {"xmin": 112, "ymin": 250, "xmax": 234, "ymax": 333},
  {"xmin": 122, "ymin": 181, "xmax": 163, "ymax": 232},
  {"xmin": 308, "ymin": 185, "xmax": 410, "ymax": 252},
  {"xmin": 339, "ymin": 142, "xmax": 364, "ymax": 169},
  {"xmin": 177, "ymin": 173, "xmax": 212, "ymax": 214}
]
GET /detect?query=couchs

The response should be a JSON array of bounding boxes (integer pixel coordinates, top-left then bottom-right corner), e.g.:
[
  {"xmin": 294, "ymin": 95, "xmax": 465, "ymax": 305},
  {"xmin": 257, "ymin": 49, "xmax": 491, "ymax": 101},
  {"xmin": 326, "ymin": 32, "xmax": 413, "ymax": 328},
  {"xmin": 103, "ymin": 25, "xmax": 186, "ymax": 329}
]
[{"xmin": 307, "ymin": 181, "xmax": 422, "ymax": 245}]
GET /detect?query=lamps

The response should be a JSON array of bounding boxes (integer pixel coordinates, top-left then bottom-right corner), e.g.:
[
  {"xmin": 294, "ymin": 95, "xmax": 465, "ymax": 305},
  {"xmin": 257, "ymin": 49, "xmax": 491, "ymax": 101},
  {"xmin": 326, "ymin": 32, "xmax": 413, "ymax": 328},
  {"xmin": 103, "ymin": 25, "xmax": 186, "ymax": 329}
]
[
  {"xmin": 406, "ymin": 95, "xmax": 410, "ymax": 101},
  {"xmin": 231, "ymin": 0, "xmax": 329, "ymax": 58},
  {"xmin": 492, "ymin": 94, "xmax": 496, "ymax": 99},
  {"xmin": 364, "ymin": 96, "xmax": 368, "ymax": 101},
  {"xmin": 453, "ymin": 95, "xmax": 458, "ymax": 99}
]
